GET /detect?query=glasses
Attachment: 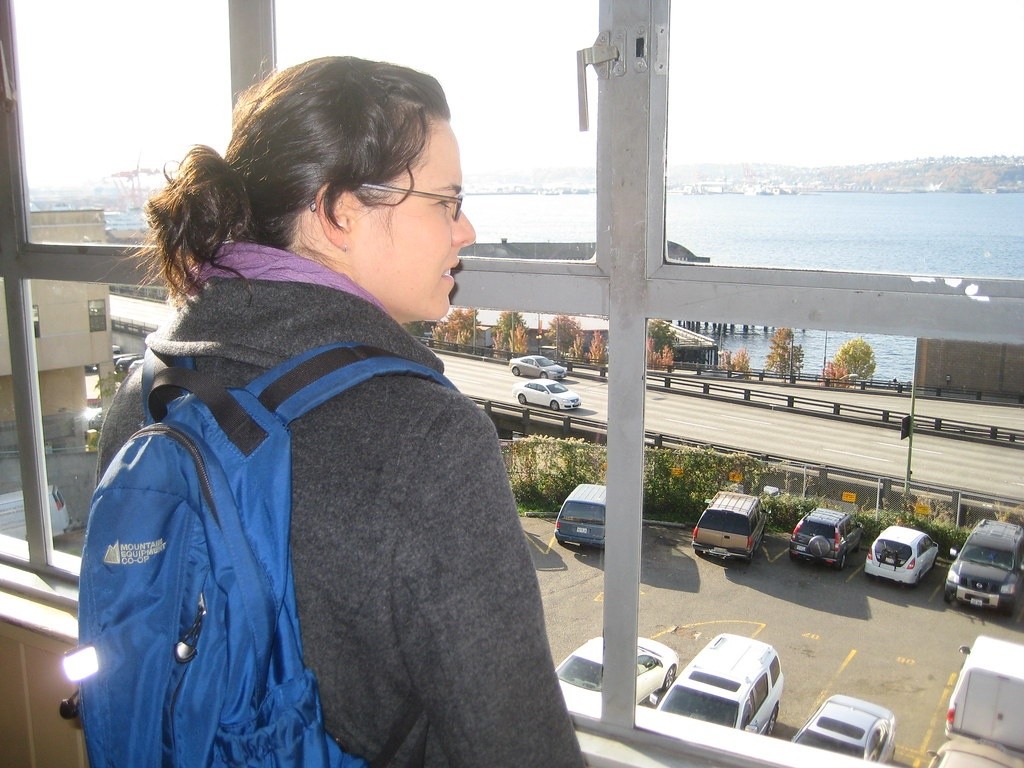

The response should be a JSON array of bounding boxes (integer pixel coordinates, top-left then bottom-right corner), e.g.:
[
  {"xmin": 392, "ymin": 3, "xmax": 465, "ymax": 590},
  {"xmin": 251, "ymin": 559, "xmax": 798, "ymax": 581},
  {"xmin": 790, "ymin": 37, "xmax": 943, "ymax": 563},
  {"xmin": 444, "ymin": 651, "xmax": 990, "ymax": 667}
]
[{"xmin": 311, "ymin": 182, "xmax": 463, "ymax": 221}]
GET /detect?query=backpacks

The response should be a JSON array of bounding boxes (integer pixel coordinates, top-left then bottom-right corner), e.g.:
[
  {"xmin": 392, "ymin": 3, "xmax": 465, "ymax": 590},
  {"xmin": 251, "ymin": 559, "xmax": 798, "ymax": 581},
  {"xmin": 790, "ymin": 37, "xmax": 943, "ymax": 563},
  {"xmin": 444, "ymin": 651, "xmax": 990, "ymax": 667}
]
[{"xmin": 79, "ymin": 339, "xmax": 368, "ymax": 768}]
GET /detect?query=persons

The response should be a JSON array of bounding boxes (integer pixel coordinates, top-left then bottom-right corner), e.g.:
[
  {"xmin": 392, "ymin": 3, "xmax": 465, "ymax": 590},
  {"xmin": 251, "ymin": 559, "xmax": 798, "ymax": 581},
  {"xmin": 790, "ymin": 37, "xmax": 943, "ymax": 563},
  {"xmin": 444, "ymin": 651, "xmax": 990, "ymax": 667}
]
[{"xmin": 97, "ymin": 55, "xmax": 584, "ymax": 768}]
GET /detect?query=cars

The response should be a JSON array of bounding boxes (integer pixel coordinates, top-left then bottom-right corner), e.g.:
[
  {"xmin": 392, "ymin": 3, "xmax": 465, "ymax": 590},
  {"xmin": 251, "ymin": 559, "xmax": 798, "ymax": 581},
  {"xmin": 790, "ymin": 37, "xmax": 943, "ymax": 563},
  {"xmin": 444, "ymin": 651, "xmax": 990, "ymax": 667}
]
[
  {"xmin": 552, "ymin": 634, "xmax": 681, "ymax": 704},
  {"xmin": 517, "ymin": 378, "xmax": 581, "ymax": 411},
  {"xmin": 863, "ymin": 525, "xmax": 940, "ymax": 587},
  {"xmin": 509, "ymin": 355, "xmax": 567, "ymax": 382},
  {"xmin": 926, "ymin": 738, "xmax": 1024, "ymax": 768},
  {"xmin": 789, "ymin": 695, "xmax": 898, "ymax": 766},
  {"xmin": 88, "ymin": 343, "xmax": 148, "ymax": 374}
]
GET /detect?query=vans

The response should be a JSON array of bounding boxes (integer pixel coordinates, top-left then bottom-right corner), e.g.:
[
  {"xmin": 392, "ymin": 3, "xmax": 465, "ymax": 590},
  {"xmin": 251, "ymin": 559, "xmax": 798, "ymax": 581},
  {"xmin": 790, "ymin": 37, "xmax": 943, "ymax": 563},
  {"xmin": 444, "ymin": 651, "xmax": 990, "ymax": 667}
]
[
  {"xmin": 942, "ymin": 634, "xmax": 1024, "ymax": 760},
  {"xmin": 651, "ymin": 633, "xmax": 784, "ymax": 737}
]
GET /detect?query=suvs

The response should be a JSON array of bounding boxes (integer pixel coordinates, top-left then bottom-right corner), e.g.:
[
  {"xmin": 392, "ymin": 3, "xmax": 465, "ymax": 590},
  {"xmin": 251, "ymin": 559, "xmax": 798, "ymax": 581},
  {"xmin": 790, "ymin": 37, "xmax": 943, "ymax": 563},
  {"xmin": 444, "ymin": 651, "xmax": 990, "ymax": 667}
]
[
  {"xmin": 942, "ymin": 520, "xmax": 1024, "ymax": 616},
  {"xmin": 789, "ymin": 507, "xmax": 863, "ymax": 571},
  {"xmin": 554, "ymin": 483, "xmax": 607, "ymax": 549},
  {"xmin": 691, "ymin": 491, "xmax": 770, "ymax": 564}
]
